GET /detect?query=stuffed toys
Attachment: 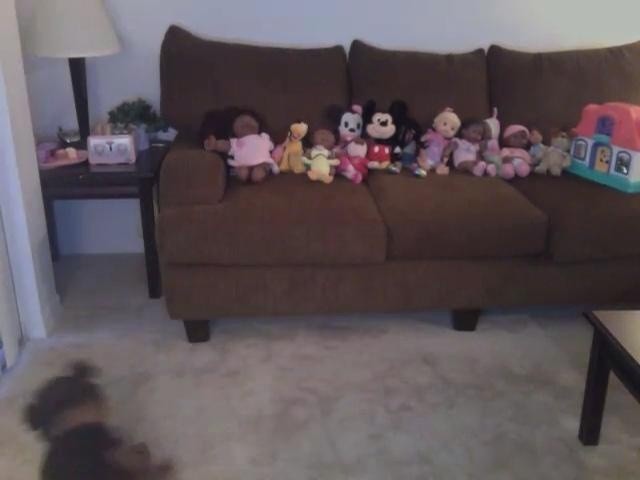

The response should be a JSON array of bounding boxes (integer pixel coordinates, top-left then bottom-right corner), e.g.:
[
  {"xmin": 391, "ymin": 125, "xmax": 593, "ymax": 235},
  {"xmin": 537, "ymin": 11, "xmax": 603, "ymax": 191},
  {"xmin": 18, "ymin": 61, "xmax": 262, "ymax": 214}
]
[
  {"xmin": 202, "ymin": 104, "xmax": 572, "ymax": 184},
  {"xmin": 203, "ymin": 103, "xmax": 574, "ymax": 185}
]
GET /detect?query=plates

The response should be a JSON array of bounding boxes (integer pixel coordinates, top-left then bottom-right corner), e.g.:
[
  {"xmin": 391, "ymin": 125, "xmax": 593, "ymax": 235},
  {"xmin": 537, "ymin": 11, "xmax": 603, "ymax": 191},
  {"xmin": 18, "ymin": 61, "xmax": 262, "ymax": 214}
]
[{"xmin": 41, "ymin": 148, "xmax": 88, "ymax": 168}]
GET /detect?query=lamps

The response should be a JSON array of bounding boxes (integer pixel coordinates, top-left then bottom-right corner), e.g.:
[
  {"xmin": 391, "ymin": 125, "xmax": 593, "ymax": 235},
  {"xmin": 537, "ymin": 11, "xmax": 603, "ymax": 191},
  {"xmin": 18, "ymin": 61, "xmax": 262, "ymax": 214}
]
[{"xmin": 30, "ymin": 0, "xmax": 126, "ymax": 139}]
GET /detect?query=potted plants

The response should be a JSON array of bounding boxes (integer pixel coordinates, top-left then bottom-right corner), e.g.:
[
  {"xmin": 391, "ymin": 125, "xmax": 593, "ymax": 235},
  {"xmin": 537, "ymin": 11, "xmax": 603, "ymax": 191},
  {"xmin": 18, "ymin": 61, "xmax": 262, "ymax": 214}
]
[{"xmin": 107, "ymin": 102, "xmax": 157, "ymax": 151}]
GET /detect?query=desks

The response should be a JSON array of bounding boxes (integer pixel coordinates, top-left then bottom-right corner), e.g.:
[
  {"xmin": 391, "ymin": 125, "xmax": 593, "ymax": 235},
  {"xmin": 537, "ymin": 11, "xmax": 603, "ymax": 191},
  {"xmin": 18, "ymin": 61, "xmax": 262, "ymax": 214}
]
[{"xmin": 37, "ymin": 134, "xmax": 161, "ymax": 300}]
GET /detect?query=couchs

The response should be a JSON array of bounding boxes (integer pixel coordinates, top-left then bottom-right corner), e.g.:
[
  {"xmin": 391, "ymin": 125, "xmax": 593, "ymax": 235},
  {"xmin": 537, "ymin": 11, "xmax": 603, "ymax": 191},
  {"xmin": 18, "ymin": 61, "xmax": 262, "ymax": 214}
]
[{"xmin": 157, "ymin": 21, "xmax": 640, "ymax": 343}]
